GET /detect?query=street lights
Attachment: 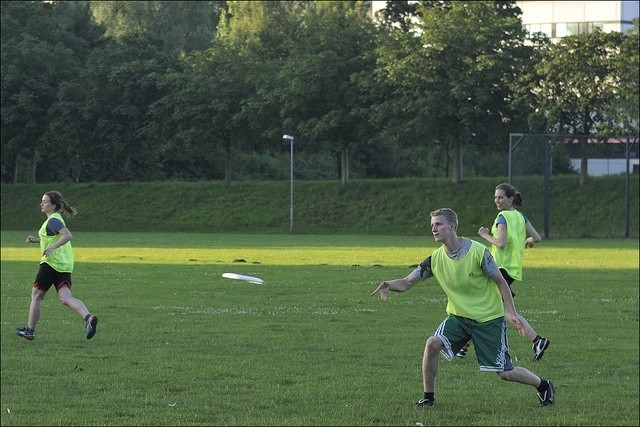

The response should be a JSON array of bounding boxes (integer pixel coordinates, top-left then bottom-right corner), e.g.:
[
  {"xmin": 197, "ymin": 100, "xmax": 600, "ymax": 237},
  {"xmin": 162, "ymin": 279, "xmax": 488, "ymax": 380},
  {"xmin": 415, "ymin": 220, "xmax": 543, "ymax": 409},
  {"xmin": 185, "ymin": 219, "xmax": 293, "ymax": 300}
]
[{"xmin": 282, "ymin": 134, "xmax": 294, "ymax": 232}]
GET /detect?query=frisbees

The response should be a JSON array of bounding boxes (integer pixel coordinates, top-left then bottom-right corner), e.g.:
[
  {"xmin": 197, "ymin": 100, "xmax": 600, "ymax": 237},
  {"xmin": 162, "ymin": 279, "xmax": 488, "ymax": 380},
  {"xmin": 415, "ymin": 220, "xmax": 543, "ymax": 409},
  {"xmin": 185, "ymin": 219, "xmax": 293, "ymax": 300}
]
[{"xmin": 221, "ymin": 272, "xmax": 264, "ymax": 285}]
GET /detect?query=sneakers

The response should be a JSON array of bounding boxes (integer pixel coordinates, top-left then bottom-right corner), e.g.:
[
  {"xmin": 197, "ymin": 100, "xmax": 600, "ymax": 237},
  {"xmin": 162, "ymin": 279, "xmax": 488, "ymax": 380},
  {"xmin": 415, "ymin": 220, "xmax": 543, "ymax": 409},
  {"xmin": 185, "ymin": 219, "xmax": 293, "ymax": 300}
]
[
  {"xmin": 16, "ymin": 327, "xmax": 35, "ymax": 340},
  {"xmin": 536, "ymin": 380, "xmax": 555, "ymax": 407},
  {"xmin": 532, "ymin": 337, "xmax": 550, "ymax": 360},
  {"xmin": 456, "ymin": 347, "xmax": 467, "ymax": 358},
  {"xmin": 416, "ymin": 398, "xmax": 435, "ymax": 407},
  {"xmin": 85, "ymin": 315, "xmax": 98, "ymax": 339}
]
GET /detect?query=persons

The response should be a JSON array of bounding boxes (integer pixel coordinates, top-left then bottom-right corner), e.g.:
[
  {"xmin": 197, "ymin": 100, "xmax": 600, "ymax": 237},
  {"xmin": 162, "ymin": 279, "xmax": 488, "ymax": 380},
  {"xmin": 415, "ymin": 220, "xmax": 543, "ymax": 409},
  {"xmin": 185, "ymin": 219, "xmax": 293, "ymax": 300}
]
[
  {"xmin": 371, "ymin": 208, "xmax": 557, "ymax": 409},
  {"xmin": 456, "ymin": 183, "xmax": 551, "ymax": 362},
  {"xmin": 15, "ymin": 191, "xmax": 99, "ymax": 340}
]
